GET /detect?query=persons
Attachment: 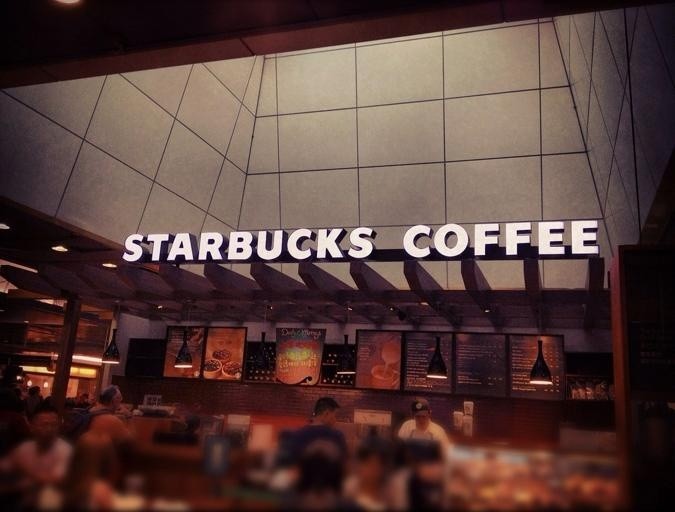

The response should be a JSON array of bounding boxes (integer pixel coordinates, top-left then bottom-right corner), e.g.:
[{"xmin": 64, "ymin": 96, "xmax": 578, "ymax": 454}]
[
  {"xmin": 0, "ymin": 384, "xmax": 89, "ymax": 417},
  {"xmin": 49, "ymin": 430, "xmax": 467, "ymax": 510},
  {"xmin": 0, "ymin": 398, "xmax": 77, "ymax": 511},
  {"xmin": 68, "ymin": 384, "xmax": 136, "ymax": 469},
  {"xmin": 275, "ymin": 395, "xmax": 350, "ymax": 465},
  {"xmin": 396, "ymin": 396, "xmax": 453, "ymax": 445}
]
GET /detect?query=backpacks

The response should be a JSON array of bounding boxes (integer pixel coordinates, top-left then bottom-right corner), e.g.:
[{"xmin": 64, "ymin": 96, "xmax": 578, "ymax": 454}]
[{"xmin": 59, "ymin": 405, "xmax": 112, "ymax": 442}]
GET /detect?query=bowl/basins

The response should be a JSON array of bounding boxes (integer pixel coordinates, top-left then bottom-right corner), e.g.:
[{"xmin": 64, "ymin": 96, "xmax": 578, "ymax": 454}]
[
  {"xmin": 222, "ymin": 360, "xmax": 242, "ymax": 378},
  {"xmin": 136, "ymin": 405, "xmax": 176, "ymax": 416},
  {"xmin": 203, "ymin": 359, "xmax": 222, "ymax": 379},
  {"xmin": 211, "ymin": 350, "xmax": 231, "ymax": 365}
]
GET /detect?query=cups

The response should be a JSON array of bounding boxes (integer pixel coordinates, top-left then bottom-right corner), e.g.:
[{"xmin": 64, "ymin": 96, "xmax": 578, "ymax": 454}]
[
  {"xmin": 371, "ymin": 365, "xmax": 394, "ymax": 387},
  {"xmin": 452, "ymin": 400, "xmax": 473, "ymax": 437}
]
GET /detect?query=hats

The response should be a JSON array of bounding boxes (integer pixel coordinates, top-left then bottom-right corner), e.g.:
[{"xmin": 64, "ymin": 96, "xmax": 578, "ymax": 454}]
[{"xmin": 410, "ymin": 399, "xmax": 430, "ymax": 415}]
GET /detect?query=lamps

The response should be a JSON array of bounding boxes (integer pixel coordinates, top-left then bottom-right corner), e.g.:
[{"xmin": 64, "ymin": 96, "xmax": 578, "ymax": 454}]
[
  {"xmin": 530, "ymin": 339, "xmax": 554, "ymax": 386},
  {"xmin": 427, "ymin": 337, "xmax": 449, "ymax": 379},
  {"xmin": 253, "ymin": 332, "xmax": 270, "ymax": 371},
  {"xmin": 173, "ymin": 330, "xmax": 193, "ymax": 369},
  {"xmin": 336, "ymin": 334, "xmax": 357, "ymax": 375},
  {"xmin": 102, "ymin": 328, "xmax": 120, "ymax": 364}
]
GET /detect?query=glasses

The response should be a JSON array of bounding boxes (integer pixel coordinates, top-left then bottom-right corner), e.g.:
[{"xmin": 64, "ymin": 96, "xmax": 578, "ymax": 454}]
[{"xmin": 114, "ymin": 396, "xmax": 122, "ymax": 401}]
